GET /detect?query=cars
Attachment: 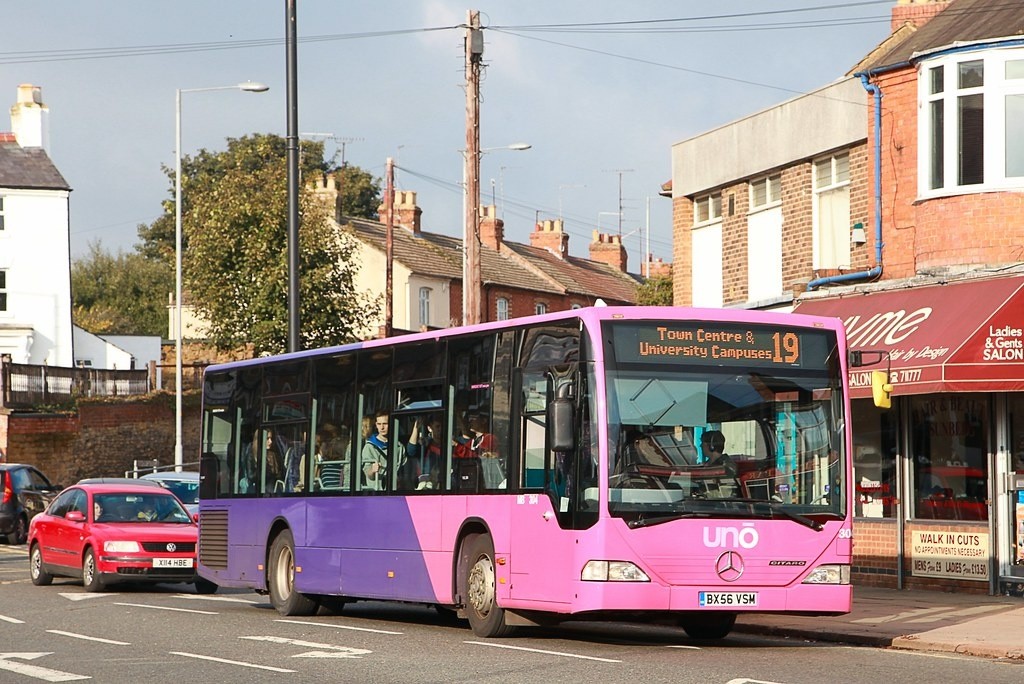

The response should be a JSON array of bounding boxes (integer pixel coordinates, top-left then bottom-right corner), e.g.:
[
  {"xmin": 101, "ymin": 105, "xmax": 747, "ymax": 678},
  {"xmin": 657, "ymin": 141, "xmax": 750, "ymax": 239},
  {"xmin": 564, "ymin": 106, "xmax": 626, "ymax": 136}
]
[
  {"xmin": 25, "ymin": 481, "xmax": 219, "ymax": 593},
  {"xmin": 138, "ymin": 471, "xmax": 199, "ymax": 527},
  {"xmin": 0, "ymin": 462, "xmax": 63, "ymax": 544}
]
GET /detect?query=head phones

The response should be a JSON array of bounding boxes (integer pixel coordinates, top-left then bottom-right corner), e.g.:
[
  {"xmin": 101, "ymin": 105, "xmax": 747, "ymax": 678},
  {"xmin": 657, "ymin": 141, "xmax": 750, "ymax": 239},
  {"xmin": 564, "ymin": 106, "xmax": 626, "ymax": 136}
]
[{"xmin": 708, "ymin": 435, "xmax": 715, "ymax": 452}]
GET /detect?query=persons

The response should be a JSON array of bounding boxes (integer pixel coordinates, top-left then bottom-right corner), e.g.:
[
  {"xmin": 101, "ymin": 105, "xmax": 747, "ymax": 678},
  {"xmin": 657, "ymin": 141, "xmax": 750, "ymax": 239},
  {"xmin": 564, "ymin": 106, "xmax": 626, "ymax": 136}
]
[
  {"xmin": 691, "ymin": 431, "xmax": 737, "ymax": 499},
  {"xmin": 94, "ymin": 498, "xmax": 125, "ymax": 521},
  {"xmin": 131, "ymin": 497, "xmax": 160, "ymax": 523},
  {"xmin": 237, "ymin": 400, "xmax": 500, "ymax": 492}
]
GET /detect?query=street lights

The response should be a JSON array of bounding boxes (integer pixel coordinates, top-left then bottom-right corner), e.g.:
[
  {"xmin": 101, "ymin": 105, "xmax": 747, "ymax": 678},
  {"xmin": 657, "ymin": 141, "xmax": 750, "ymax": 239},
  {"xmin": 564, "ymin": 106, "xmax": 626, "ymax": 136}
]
[
  {"xmin": 461, "ymin": 145, "xmax": 530, "ymax": 325},
  {"xmin": 173, "ymin": 81, "xmax": 272, "ymax": 470}
]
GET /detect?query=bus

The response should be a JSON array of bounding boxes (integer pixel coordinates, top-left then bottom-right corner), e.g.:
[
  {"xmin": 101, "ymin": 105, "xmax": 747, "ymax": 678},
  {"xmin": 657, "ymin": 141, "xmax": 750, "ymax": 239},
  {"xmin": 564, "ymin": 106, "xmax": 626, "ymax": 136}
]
[{"xmin": 197, "ymin": 304, "xmax": 893, "ymax": 637}]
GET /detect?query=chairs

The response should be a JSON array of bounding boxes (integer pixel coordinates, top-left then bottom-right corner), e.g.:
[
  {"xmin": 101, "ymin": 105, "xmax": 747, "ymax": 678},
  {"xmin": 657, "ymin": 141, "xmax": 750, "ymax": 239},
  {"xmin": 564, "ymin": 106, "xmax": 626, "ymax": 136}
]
[{"xmin": 240, "ymin": 442, "xmax": 505, "ymax": 494}]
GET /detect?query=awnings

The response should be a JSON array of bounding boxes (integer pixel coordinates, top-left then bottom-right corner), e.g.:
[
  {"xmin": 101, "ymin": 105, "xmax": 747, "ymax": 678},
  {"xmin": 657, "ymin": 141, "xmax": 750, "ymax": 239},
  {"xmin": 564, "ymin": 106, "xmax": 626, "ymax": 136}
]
[{"xmin": 775, "ymin": 277, "xmax": 1024, "ymax": 401}]
